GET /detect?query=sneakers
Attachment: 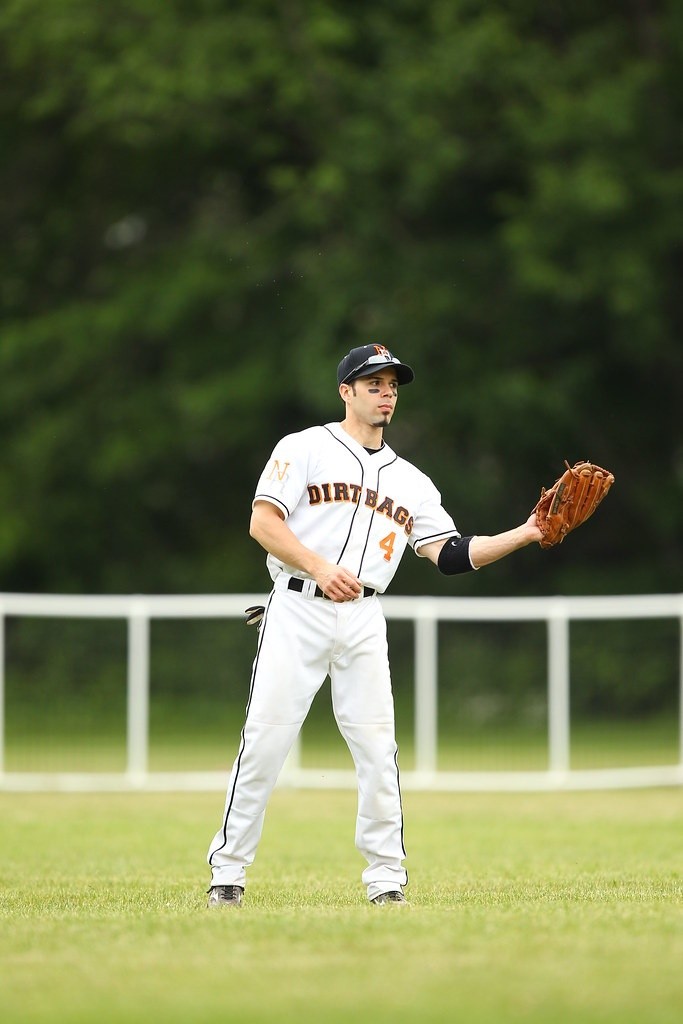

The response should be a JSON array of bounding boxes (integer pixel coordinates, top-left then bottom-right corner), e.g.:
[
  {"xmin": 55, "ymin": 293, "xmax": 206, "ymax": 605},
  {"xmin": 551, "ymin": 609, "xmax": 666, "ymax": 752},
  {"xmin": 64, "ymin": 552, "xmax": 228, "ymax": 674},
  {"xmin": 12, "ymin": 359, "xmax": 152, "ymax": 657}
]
[
  {"xmin": 206, "ymin": 885, "xmax": 245, "ymax": 908},
  {"xmin": 369, "ymin": 891, "xmax": 408, "ymax": 906}
]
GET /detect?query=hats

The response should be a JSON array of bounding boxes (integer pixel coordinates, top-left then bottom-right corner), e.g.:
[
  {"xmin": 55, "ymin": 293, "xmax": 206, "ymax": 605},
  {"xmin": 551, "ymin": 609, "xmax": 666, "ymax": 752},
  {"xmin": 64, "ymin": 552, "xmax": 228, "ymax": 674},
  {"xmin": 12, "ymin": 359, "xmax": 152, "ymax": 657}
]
[{"xmin": 337, "ymin": 342, "xmax": 416, "ymax": 387}]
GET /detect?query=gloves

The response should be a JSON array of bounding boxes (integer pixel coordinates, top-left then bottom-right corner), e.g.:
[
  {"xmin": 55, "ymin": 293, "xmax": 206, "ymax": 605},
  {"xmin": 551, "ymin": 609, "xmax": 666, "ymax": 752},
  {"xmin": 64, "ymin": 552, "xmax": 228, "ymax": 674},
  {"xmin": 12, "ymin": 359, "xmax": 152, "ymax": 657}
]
[{"xmin": 245, "ymin": 605, "xmax": 265, "ymax": 632}]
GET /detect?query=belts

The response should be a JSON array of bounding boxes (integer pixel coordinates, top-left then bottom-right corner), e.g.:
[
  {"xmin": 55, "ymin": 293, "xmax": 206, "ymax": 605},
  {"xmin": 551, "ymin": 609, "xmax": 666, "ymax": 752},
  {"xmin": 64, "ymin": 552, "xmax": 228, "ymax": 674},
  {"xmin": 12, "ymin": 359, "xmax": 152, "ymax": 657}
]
[{"xmin": 288, "ymin": 577, "xmax": 376, "ymax": 600}]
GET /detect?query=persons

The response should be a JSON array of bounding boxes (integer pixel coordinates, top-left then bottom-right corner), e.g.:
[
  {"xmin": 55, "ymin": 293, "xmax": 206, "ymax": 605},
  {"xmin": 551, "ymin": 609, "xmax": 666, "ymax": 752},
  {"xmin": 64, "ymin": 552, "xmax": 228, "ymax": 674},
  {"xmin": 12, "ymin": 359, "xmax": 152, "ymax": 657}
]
[{"xmin": 207, "ymin": 345, "xmax": 545, "ymax": 907}]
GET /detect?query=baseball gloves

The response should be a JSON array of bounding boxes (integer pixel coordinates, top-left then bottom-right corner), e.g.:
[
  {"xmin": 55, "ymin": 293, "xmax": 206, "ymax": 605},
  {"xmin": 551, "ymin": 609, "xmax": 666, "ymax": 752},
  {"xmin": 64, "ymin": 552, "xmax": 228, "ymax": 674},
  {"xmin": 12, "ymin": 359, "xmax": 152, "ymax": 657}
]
[{"xmin": 531, "ymin": 460, "xmax": 615, "ymax": 551}]
